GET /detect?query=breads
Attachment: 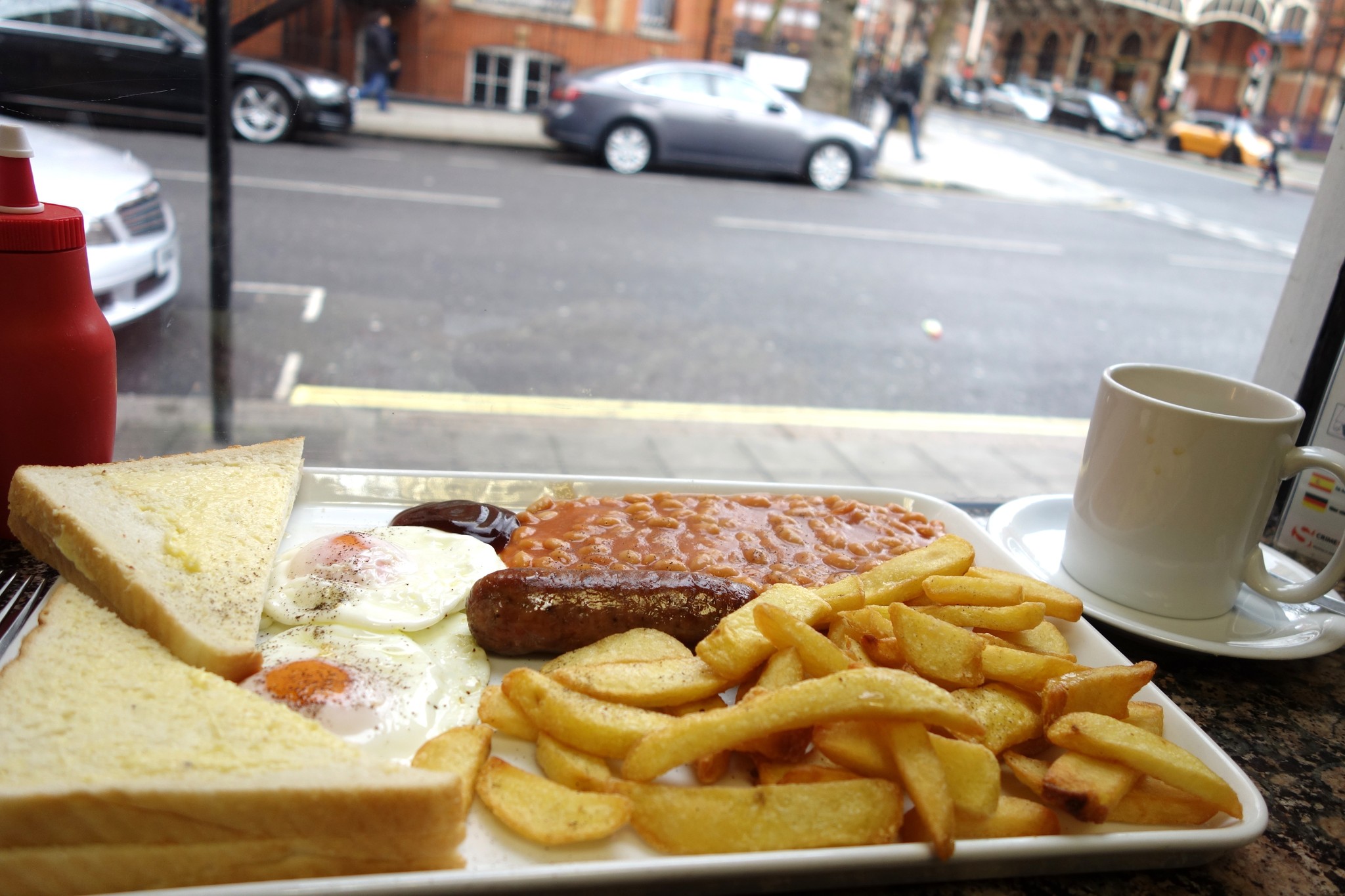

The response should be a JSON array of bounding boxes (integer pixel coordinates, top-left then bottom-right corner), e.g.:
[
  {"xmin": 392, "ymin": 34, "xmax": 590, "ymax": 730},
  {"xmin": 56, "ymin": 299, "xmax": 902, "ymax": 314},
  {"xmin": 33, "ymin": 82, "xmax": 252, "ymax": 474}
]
[{"xmin": 2, "ymin": 432, "xmax": 466, "ymax": 896}]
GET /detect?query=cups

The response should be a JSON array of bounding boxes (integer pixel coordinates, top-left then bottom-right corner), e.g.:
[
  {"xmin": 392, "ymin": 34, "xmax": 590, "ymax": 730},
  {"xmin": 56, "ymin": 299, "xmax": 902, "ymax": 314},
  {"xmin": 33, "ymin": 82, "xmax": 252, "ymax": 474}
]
[{"xmin": 1061, "ymin": 363, "xmax": 1344, "ymax": 619}]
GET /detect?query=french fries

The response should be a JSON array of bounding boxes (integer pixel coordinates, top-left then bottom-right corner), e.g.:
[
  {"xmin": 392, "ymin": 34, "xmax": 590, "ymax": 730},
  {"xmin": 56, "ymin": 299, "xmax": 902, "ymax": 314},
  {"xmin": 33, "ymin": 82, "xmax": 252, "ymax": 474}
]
[{"xmin": 412, "ymin": 531, "xmax": 1246, "ymax": 855}]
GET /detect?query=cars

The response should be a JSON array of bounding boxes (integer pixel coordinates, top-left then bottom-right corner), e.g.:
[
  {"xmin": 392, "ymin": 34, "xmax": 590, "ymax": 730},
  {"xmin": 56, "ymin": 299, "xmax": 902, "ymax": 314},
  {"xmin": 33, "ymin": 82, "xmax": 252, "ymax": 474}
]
[
  {"xmin": 0, "ymin": 0, "xmax": 359, "ymax": 144},
  {"xmin": 0, "ymin": 113, "xmax": 180, "ymax": 328},
  {"xmin": 539, "ymin": 56, "xmax": 883, "ymax": 194},
  {"xmin": 853, "ymin": 43, "xmax": 1058, "ymax": 122},
  {"xmin": 1051, "ymin": 88, "xmax": 1148, "ymax": 142},
  {"xmin": 1166, "ymin": 108, "xmax": 1273, "ymax": 167}
]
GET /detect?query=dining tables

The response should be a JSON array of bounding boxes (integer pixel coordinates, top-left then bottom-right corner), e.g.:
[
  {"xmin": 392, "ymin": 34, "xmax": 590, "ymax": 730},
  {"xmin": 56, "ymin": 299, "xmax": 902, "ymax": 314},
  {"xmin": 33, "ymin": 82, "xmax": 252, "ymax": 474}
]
[{"xmin": 2, "ymin": 518, "xmax": 1345, "ymax": 896}]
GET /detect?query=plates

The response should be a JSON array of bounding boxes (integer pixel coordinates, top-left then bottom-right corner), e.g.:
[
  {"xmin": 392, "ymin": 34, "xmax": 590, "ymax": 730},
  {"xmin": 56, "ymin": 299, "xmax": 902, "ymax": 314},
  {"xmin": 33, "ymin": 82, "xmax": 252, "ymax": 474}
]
[
  {"xmin": 1, "ymin": 468, "xmax": 1270, "ymax": 894},
  {"xmin": 985, "ymin": 493, "xmax": 1345, "ymax": 658}
]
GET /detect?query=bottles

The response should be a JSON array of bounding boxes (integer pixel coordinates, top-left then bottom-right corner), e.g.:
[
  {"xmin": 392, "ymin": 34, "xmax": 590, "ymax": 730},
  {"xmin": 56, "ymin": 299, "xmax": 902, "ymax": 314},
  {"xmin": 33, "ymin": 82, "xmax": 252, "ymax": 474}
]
[{"xmin": 0, "ymin": 124, "xmax": 117, "ymax": 537}]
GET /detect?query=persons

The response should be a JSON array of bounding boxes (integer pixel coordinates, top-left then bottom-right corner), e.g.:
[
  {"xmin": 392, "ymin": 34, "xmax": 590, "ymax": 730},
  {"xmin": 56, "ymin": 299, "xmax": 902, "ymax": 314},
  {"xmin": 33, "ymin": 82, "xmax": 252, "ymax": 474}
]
[
  {"xmin": 353, "ymin": 11, "xmax": 401, "ymax": 111},
  {"xmin": 876, "ymin": 43, "xmax": 930, "ymax": 159},
  {"xmin": 1251, "ymin": 115, "xmax": 1293, "ymax": 189}
]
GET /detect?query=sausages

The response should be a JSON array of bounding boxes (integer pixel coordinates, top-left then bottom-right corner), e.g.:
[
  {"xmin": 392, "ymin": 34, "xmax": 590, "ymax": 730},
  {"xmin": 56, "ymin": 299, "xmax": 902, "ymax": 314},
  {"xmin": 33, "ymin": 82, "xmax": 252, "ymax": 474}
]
[{"xmin": 466, "ymin": 566, "xmax": 762, "ymax": 660}]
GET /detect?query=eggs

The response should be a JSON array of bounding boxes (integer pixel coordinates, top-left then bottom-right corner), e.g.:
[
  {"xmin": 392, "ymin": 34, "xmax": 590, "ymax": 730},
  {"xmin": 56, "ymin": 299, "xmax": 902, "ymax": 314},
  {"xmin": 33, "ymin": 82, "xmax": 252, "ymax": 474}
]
[{"xmin": 237, "ymin": 523, "xmax": 507, "ymax": 770}]
